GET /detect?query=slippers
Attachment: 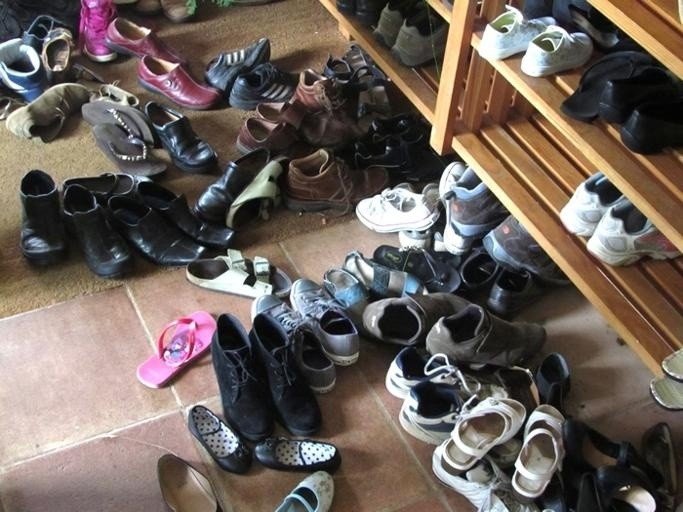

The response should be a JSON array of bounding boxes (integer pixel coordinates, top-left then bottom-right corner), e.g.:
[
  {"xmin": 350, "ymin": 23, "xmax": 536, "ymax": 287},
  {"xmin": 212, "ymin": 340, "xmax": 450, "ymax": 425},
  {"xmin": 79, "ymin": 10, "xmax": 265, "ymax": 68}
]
[
  {"xmin": 184, "ymin": 248, "xmax": 292, "ymax": 298},
  {"xmin": 135, "ymin": 309, "xmax": 218, "ymax": 389},
  {"xmin": 81, "ymin": 79, "xmax": 167, "ymax": 176}
]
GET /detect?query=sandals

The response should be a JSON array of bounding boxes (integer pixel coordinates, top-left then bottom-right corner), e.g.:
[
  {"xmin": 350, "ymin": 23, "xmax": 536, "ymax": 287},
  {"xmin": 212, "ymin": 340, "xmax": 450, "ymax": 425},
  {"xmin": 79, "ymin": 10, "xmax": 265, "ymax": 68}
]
[{"xmin": 443, "ymin": 394, "xmax": 565, "ymax": 499}]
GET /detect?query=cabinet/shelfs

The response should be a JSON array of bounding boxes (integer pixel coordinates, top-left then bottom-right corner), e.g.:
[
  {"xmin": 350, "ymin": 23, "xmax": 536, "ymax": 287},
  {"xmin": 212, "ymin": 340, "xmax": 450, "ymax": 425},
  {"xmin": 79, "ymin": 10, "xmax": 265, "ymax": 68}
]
[{"xmin": 314, "ymin": 1, "xmax": 682, "ymax": 378}]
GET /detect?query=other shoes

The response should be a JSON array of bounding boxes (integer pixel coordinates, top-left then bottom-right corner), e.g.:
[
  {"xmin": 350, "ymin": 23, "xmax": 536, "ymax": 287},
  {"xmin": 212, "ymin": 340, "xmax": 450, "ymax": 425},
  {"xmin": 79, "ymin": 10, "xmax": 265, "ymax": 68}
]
[
  {"xmin": 334, "ymin": 0, "xmax": 450, "ymax": 66},
  {"xmin": 204, "ymin": 37, "xmax": 431, "ymax": 228}
]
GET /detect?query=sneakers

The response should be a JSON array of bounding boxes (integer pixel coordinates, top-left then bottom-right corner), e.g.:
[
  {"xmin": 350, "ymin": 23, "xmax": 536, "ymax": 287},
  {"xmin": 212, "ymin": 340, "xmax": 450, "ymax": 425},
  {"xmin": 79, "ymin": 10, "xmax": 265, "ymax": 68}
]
[
  {"xmin": 560, "ymin": 172, "xmax": 628, "ymax": 238},
  {"xmin": 78, "ymin": 1, "xmax": 189, "ymax": 66},
  {"xmin": 145, "ymin": 102, "xmax": 216, "ymax": 172},
  {"xmin": 20, "ymin": 170, "xmax": 68, "ymax": 263},
  {"xmin": 207, "ymin": 314, "xmax": 326, "ymax": 442},
  {"xmin": 273, "ymin": 470, "xmax": 334, "ymax": 511},
  {"xmin": 156, "ymin": 452, "xmax": 223, "ymax": 511},
  {"xmin": 187, "ymin": 404, "xmax": 252, "ymax": 473},
  {"xmin": 317, "ymin": 162, "xmax": 571, "ymax": 442},
  {"xmin": 61, "ymin": 173, "xmax": 234, "ymax": 281},
  {"xmin": 586, "ymin": 198, "xmax": 682, "ymax": 266},
  {"xmin": 479, "ymin": 1, "xmax": 682, "ymax": 156},
  {"xmin": 252, "ymin": 278, "xmax": 360, "ymax": 393},
  {"xmin": 140, "ymin": 55, "xmax": 223, "ymax": 109},
  {"xmin": 433, "ymin": 421, "xmax": 683, "ymax": 512},
  {"xmin": 253, "ymin": 438, "xmax": 341, "ymax": 473},
  {"xmin": 0, "ymin": 1, "xmax": 104, "ymax": 146}
]
